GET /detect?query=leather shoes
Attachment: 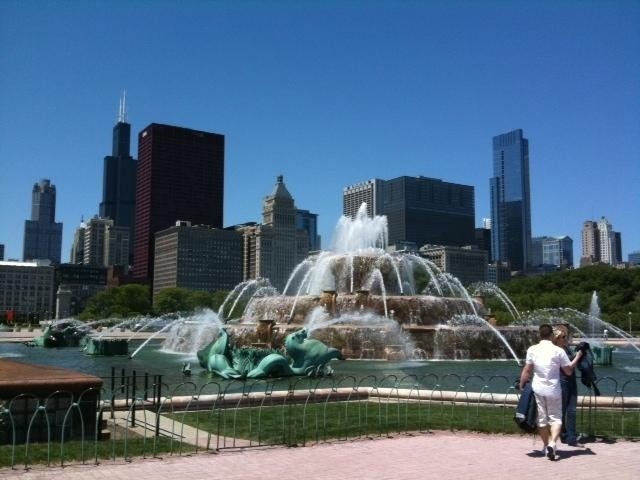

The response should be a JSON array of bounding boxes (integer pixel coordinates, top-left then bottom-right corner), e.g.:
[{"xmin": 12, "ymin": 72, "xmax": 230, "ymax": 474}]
[{"xmin": 560, "ymin": 439, "xmax": 584, "ymax": 447}]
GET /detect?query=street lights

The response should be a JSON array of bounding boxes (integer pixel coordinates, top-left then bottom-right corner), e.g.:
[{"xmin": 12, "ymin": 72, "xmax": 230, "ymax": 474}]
[{"xmin": 629, "ymin": 312, "xmax": 631, "ymax": 333}]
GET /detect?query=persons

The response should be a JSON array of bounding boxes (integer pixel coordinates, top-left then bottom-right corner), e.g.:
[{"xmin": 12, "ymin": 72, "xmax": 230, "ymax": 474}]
[
  {"xmin": 603, "ymin": 328, "xmax": 609, "ymax": 341},
  {"xmin": 547, "ymin": 325, "xmax": 583, "ymax": 446},
  {"xmin": 518, "ymin": 324, "xmax": 583, "ymax": 461}
]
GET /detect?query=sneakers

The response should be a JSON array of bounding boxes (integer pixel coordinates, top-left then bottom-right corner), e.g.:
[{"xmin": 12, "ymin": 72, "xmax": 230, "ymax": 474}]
[{"xmin": 542, "ymin": 441, "xmax": 556, "ymax": 459}]
